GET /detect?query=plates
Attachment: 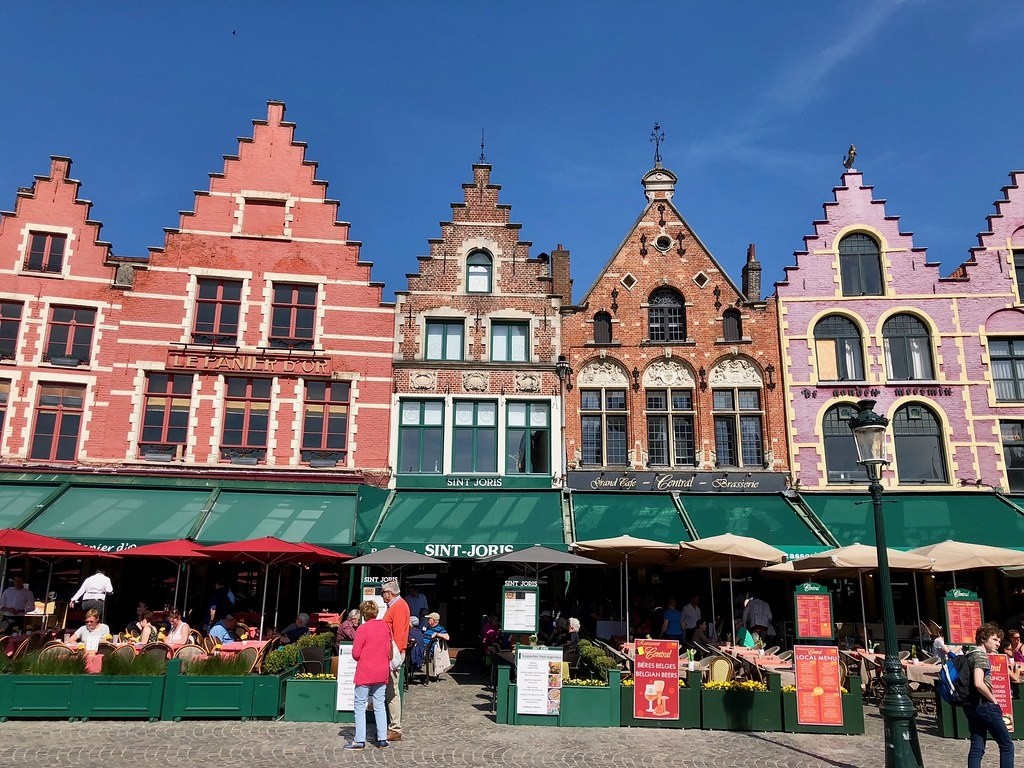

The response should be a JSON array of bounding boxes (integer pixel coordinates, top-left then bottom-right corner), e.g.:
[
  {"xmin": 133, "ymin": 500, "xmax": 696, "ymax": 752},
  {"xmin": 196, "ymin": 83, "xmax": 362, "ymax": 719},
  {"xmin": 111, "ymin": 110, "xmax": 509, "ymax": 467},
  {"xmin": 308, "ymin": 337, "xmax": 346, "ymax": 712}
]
[
  {"xmin": 548, "ymin": 689, "xmax": 560, "ymax": 699},
  {"xmin": 652, "ymin": 711, "xmax": 669, "ymax": 715}
]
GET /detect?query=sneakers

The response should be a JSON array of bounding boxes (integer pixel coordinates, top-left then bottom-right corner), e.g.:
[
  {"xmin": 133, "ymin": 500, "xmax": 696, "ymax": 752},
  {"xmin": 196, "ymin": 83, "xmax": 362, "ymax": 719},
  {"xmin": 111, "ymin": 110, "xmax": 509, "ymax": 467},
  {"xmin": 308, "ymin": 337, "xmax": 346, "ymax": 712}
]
[
  {"xmin": 344, "ymin": 740, "xmax": 365, "ymax": 751},
  {"xmin": 378, "ymin": 741, "xmax": 389, "ymax": 749},
  {"xmin": 387, "ymin": 730, "xmax": 401, "ymax": 741}
]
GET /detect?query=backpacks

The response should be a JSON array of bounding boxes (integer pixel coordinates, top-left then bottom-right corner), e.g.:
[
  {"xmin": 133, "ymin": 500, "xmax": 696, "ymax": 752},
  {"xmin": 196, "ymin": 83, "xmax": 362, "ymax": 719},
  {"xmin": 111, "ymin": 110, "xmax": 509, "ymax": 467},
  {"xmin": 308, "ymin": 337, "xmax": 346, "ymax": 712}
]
[{"xmin": 937, "ymin": 651, "xmax": 987, "ymax": 707}]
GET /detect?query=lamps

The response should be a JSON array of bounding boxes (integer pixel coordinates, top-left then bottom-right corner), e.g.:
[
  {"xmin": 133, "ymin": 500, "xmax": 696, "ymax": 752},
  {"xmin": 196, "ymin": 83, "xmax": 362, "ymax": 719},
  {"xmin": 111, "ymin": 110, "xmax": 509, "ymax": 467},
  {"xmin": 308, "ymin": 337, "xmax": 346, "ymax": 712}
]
[
  {"xmin": 600, "ymin": 471, "xmax": 605, "ymax": 476},
  {"xmin": 692, "ymin": 472, "xmax": 697, "ymax": 477},
  {"xmin": 745, "ymin": 472, "xmax": 752, "ymax": 477},
  {"xmin": 723, "ymin": 472, "xmax": 729, "ymax": 478},
  {"xmin": 624, "ymin": 471, "xmax": 628, "ymax": 476},
  {"xmin": 655, "ymin": 472, "xmax": 659, "ymax": 477}
]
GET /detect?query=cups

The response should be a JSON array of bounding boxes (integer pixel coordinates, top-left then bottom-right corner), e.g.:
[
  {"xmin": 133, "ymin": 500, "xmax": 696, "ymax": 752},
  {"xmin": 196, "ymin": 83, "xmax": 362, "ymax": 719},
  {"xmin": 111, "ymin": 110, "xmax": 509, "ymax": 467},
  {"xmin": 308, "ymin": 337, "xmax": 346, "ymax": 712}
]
[
  {"xmin": 64, "ymin": 634, "xmax": 70, "ymax": 643},
  {"xmin": 164, "ymin": 607, "xmax": 169, "ymax": 611},
  {"xmin": 113, "ymin": 635, "xmax": 119, "ymax": 643},
  {"xmin": 654, "ymin": 681, "xmax": 664, "ymax": 704},
  {"xmin": 1009, "ymin": 658, "xmax": 1014, "ymax": 663}
]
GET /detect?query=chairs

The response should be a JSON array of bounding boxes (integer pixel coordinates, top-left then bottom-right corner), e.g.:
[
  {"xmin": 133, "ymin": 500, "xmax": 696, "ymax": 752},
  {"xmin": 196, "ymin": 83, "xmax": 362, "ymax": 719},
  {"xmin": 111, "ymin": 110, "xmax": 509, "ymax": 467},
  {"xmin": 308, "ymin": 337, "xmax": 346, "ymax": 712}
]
[{"xmin": 0, "ymin": 607, "xmax": 1024, "ymax": 689}]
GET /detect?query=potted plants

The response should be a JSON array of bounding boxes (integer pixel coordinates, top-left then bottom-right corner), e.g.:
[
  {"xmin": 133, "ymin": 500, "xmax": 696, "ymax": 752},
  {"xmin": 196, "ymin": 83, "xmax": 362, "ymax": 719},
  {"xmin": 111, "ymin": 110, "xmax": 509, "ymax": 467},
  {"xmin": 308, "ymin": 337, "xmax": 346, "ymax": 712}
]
[
  {"xmin": 296, "ymin": 632, "xmax": 325, "ymax": 675},
  {"xmin": 173, "ymin": 650, "xmax": 257, "ymax": 721},
  {"xmin": 912, "ymin": 645, "xmax": 919, "ymax": 665},
  {"xmin": 81, "ymin": 651, "xmax": 168, "ymax": 724},
  {"xmin": 252, "ymin": 643, "xmax": 302, "ymax": 721},
  {"xmin": 0, "ymin": 648, "xmax": 84, "ymax": 723},
  {"xmin": 756, "ymin": 636, "xmax": 764, "ymax": 655}
]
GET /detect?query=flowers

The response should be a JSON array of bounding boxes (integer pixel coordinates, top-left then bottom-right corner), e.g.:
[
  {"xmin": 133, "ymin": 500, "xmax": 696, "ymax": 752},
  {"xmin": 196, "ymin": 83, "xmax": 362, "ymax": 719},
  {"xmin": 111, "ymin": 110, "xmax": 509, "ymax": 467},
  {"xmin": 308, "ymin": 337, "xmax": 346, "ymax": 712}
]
[
  {"xmin": 528, "ymin": 635, "xmax": 537, "ymax": 643},
  {"xmin": 158, "ymin": 632, "xmax": 165, "ymax": 641},
  {"xmin": 868, "ymin": 640, "xmax": 880, "ymax": 649},
  {"xmin": 622, "ymin": 680, "xmax": 686, "ymax": 688},
  {"xmin": 686, "ymin": 648, "xmax": 694, "ymax": 661},
  {"xmin": 703, "ymin": 680, "xmax": 769, "ymax": 691},
  {"xmin": 781, "ymin": 685, "xmax": 848, "ymax": 694},
  {"xmin": 159, "ymin": 628, "xmax": 165, "ymax": 633},
  {"xmin": 294, "ymin": 672, "xmax": 336, "ymax": 680},
  {"xmin": 104, "ymin": 634, "xmax": 113, "ymax": 641},
  {"xmin": 240, "ymin": 635, "xmax": 247, "ymax": 641},
  {"xmin": 215, "ymin": 644, "xmax": 222, "ymax": 652},
  {"xmin": 562, "ymin": 676, "xmax": 606, "ymax": 687},
  {"xmin": 123, "ymin": 633, "xmax": 132, "ymax": 640},
  {"xmin": 77, "ymin": 643, "xmax": 86, "ymax": 652}
]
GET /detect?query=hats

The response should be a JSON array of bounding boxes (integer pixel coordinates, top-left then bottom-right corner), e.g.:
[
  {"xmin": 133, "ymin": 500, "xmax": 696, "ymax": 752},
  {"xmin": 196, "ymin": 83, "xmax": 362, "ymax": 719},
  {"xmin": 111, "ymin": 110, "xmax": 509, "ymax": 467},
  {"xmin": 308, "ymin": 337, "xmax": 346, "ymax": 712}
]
[
  {"xmin": 425, "ymin": 612, "xmax": 440, "ymax": 623},
  {"xmin": 410, "ymin": 616, "xmax": 419, "ymax": 627},
  {"xmin": 539, "ymin": 611, "xmax": 551, "ymax": 617}
]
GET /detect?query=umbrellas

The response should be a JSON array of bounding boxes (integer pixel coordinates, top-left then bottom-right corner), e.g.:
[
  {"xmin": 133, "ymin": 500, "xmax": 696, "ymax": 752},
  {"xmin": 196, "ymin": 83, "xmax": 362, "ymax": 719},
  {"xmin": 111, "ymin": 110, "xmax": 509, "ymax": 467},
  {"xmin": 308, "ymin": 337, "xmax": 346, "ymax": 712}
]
[
  {"xmin": 474, "ymin": 530, "xmax": 1023, "ymax": 644},
  {"xmin": 0, "ymin": 528, "xmax": 358, "ymax": 642},
  {"xmin": 340, "ymin": 544, "xmax": 449, "ymax": 576}
]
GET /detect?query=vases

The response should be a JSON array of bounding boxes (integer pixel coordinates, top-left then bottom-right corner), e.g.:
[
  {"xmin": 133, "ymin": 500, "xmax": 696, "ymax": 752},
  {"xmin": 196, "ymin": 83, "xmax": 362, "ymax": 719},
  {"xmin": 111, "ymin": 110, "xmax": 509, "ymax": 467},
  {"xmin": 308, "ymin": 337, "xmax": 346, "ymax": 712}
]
[
  {"xmin": 242, "ymin": 640, "xmax": 247, "ymax": 645},
  {"xmin": 700, "ymin": 687, "xmax": 773, "ymax": 733},
  {"xmin": 620, "ymin": 683, "xmax": 691, "ymax": 730},
  {"xmin": 781, "ymin": 690, "xmax": 854, "ymax": 736},
  {"xmin": 107, "ymin": 641, "xmax": 110, "ymax": 643},
  {"xmin": 284, "ymin": 677, "xmax": 337, "ymax": 722},
  {"xmin": 688, "ymin": 661, "xmax": 695, "ymax": 671},
  {"xmin": 530, "ymin": 642, "xmax": 537, "ymax": 647},
  {"xmin": 126, "ymin": 640, "xmax": 128, "ymax": 644},
  {"xmin": 560, "ymin": 685, "xmax": 611, "ymax": 727},
  {"xmin": 215, "ymin": 651, "xmax": 220, "ymax": 656},
  {"xmin": 868, "ymin": 649, "xmax": 874, "ymax": 654}
]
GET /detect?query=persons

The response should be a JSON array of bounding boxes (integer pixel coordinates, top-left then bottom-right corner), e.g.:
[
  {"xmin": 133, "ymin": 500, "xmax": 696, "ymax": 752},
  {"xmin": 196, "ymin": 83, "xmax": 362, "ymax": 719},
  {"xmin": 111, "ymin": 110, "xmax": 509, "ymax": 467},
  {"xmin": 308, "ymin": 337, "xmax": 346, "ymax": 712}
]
[
  {"xmin": 208, "ymin": 579, "xmax": 234, "ymax": 628},
  {"xmin": 66, "ymin": 607, "xmax": 114, "ymax": 656},
  {"xmin": 405, "ymin": 586, "xmax": 450, "ymax": 672},
  {"xmin": 962, "ymin": 623, "xmax": 1015, "ymax": 768},
  {"xmin": 734, "ymin": 591, "xmax": 773, "ymax": 646},
  {"xmin": 336, "ymin": 581, "xmax": 411, "ymax": 752},
  {"xmin": 997, "ymin": 629, "xmax": 1024, "ymax": 699},
  {"xmin": 272, "ymin": 613, "xmax": 312, "ymax": 651},
  {"xmin": 137, "ymin": 598, "xmax": 156, "ymax": 622},
  {"xmin": 136, "ymin": 609, "xmax": 195, "ymax": 649},
  {"xmin": 0, "ymin": 574, "xmax": 35, "ymax": 636},
  {"xmin": 208, "ymin": 614, "xmax": 242, "ymax": 641},
  {"xmin": 481, "ymin": 612, "xmax": 502, "ymax": 654},
  {"xmin": 539, "ymin": 610, "xmax": 582, "ymax": 647},
  {"xmin": 658, "ymin": 594, "xmax": 714, "ymax": 658},
  {"xmin": 68, "ymin": 563, "xmax": 114, "ymax": 626}
]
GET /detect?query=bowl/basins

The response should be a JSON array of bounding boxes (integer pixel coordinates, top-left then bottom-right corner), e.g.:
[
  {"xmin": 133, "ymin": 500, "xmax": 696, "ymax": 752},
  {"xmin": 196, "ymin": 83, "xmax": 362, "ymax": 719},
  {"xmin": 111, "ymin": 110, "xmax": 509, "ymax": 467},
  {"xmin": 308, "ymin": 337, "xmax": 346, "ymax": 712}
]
[
  {"xmin": 857, "ymin": 649, "xmax": 865, "ymax": 653},
  {"xmin": 242, "ymin": 641, "xmax": 246, "ymax": 644}
]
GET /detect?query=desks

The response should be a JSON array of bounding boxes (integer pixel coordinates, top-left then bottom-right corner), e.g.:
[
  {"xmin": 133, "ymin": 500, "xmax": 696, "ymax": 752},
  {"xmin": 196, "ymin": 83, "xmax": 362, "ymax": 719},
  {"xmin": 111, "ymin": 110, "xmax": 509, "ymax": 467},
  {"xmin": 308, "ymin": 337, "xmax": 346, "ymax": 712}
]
[
  {"xmin": 134, "ymin": 644, "xmax": 186, "ymax": 659},
  {"xmin": 736, "ymin": 653, "xmax": 778, "ymax": 659},
  {"xmin": 192, "ymin": 651, "xmax": 238, "ymax": 663},
  {"xmin": 863, "ymin": 654, "xmax": 885, "ymax": 661},
  {"xmin": 757, "ymin": 660, "xmax": 791, "ymax": 682},
  {"xmin": 725, "ymin": 649, "xmax": 757, "ymax": 657},
  {"xmin": 901, "ymin": 659, "xmax": 943, "ymax": 713},
  {"xmin": 211, "ymin": 642, "xmax": 264, "ymax": 655},
  {"xmin": 307, "ymin": 612, "xmax": 340, "ymax": 623},
  {"xmin": 717, "ymin": 645, "xmax": 747, "ymax": 652},
  {"xmin": 83, "ymin": 650, "xmax": 106, "ymax": 674}
]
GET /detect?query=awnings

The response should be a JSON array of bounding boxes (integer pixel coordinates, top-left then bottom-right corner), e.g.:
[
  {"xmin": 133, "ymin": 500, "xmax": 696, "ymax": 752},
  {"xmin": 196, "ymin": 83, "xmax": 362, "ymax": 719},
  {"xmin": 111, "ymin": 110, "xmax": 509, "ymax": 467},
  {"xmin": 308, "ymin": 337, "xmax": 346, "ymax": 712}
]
[
  {"xmin": 367, "ymin": 484, "xmax": 1024, "ymax": 557},
  {"xmin": 0, "ymin": 471, "xmax": 359, "ymax": 547}
]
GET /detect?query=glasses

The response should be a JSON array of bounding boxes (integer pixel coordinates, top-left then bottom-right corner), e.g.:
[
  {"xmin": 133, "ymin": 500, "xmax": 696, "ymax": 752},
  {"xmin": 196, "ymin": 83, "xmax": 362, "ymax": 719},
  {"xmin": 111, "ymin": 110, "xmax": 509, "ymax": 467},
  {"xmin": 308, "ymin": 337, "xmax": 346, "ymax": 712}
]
[
  {"xmin": 1013, "ymin": 636, "xmax": 1020, "ymax": 639},
  {"xmin": 381, "ymin": 590, "xmax": 387, "ymax": 594},
  {"xmin": 494, "ymin": 619, "xmax": 499, "ymax": 622},
  {"xmin": 85, "ymin": 621, "xmax": 93, "ymax": 623}
]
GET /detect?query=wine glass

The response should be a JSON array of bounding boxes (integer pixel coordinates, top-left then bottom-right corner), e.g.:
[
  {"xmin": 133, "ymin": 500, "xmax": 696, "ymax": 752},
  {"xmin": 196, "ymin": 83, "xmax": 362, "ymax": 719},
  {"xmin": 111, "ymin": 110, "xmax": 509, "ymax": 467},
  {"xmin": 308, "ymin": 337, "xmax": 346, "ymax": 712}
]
[{"xmin": 644, "ymin": 685, "xmax": 657, "ymax": 712}]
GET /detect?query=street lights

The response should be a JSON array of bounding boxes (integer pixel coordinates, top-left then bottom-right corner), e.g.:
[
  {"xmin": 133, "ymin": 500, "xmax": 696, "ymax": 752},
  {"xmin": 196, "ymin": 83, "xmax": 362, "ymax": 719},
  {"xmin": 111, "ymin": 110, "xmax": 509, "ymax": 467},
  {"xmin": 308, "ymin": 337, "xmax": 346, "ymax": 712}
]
[{"xmin": 845, "ymin": 399, "xmax": 924, "ymax": 768}]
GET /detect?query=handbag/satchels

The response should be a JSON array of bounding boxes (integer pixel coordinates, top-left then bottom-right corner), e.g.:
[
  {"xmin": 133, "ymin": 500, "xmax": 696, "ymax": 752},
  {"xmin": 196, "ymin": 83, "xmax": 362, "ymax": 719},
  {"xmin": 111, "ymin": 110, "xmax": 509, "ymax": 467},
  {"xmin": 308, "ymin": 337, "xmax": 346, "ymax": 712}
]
[
  {"xmin": 434, "ymin": 638, "xmax": 451, "ymax": 676},
  {"xmin": 390, "ymin": 625, "xmax": 402, "ymax": 671}
]
